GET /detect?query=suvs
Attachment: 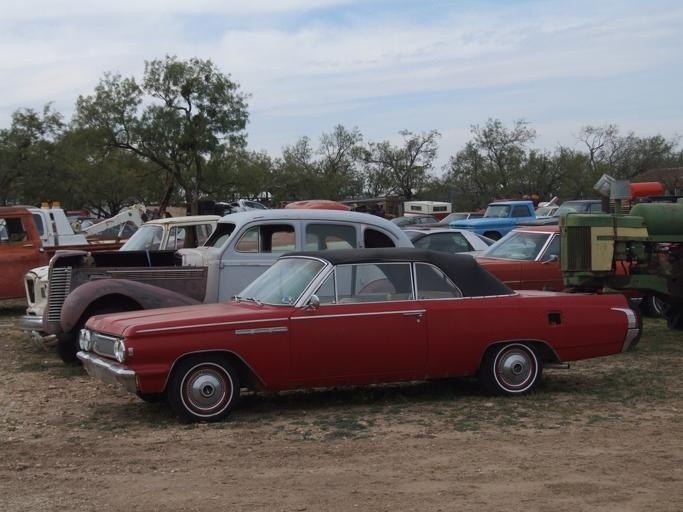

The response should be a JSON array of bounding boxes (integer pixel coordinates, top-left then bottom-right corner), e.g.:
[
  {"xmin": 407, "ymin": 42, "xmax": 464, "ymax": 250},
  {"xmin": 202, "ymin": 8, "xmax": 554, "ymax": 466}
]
[{"xmin": 558, "ymin": 193, "xmax": 683, "ymax": 319}]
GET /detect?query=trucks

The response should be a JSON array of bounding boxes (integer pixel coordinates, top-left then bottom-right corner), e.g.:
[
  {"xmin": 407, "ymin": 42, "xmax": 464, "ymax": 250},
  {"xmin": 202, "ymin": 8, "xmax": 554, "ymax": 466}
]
[
  {"xmin": 449, "ymin": 200, "xmax": 559, "ymax": 241},
  {"xmin": 44, "ymin": 208, "xmax": 405, "ymax": 366}
]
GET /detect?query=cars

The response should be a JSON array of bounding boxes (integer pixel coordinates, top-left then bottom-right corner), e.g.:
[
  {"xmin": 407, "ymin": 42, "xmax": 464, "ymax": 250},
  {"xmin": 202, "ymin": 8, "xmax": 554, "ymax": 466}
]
[
  {"xmin": 472, "ymin": 225, "xmax": 565, "ymax": 293},
  {"xmin": 0, "ymin": 199, "xmax": 354, "ymax": 300},
  {"xmin": 389, "ymin": 215, "xmax": 439, "ymax": 228},
  {"xmin": 442, "ymin": 212, "xmax": 485, "ymax": 224},
  {"xmin": 76, "ymin": 246, "xmax": 642, "ymax": 420},
  {"xmin": 402, "ymin": 227, "xmax": 496, "ymax": 253},
  {"xmin": 18, "ymin": 215, "xmax": 221, "ymax": 342},
  {"xmin": 533, "ymin": 197, "xmax": 604, "ymax": 217}
]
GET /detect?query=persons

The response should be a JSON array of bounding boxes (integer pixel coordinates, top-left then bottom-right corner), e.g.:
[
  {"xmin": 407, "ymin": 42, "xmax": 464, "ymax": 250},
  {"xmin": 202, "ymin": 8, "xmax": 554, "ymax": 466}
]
[{"xmin": 140, "ymin": 206, "xmax": 172, "ymax": 222}]
